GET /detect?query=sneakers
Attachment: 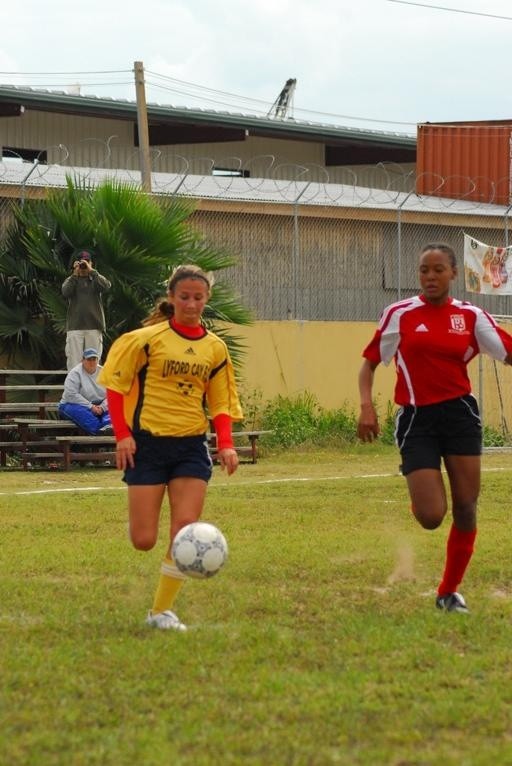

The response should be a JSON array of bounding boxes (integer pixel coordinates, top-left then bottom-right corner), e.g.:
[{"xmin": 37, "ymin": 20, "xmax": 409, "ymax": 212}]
[
  {"xmin": 144, "ymin": 608, "xmax": 189, "ymax": 632},
  {"xmin": 99, "ymin": 423, "xmax": 113, "ymax": 435},
  {"xmin": 435, "ymin": 589, "xmax": 471, "ymax": 618}
]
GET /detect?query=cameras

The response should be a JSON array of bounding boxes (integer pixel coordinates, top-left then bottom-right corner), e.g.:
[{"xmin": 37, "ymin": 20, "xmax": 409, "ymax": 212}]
[{"xmin": 80, "ymin": 263, "xmax": 87, "ymax": 269}]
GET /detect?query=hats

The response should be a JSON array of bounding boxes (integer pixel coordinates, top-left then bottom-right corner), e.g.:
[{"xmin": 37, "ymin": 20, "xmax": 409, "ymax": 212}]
[
  {"xmin": 75, "ymin": 250, "xmax": 91, "ymax": 260},
  {"xmin": 82, "ymin": 348, "xmax": 100, "ymax": 360}
]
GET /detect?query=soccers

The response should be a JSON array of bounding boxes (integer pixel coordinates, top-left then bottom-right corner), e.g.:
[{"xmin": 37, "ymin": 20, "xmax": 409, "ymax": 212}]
[{"xmin": 172, "ymin": 522, "xmax": 228, "ymax": 580}]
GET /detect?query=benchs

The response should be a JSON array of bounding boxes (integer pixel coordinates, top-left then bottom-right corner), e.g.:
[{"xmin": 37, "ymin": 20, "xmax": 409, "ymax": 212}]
[{"xmin": 0, "ymin": 370, "xmax": 270, "ymax": 472}]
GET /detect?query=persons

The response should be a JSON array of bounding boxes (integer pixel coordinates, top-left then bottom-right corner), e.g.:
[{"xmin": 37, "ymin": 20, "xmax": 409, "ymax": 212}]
[
  {"xmin": 62, "ymin": 251, "xmax": 112, "ymax": 370},
  {"xmin": 98, "ymin": 265, "xmax": 243, "ymax": 632},
  {"xmin": 356, "ymin": 242, "xmax": 512, "ymax": 616},
  {"xmin": 59, "ymin": 349, "xmax": 113, "ymax": 434}
]
